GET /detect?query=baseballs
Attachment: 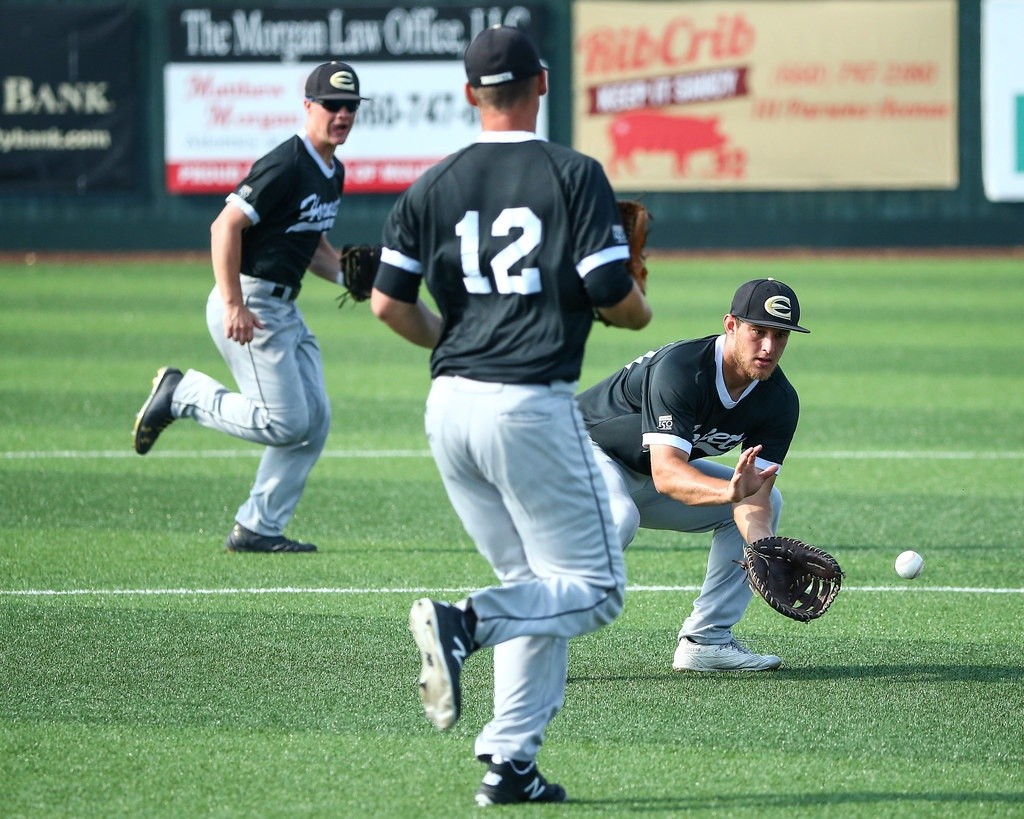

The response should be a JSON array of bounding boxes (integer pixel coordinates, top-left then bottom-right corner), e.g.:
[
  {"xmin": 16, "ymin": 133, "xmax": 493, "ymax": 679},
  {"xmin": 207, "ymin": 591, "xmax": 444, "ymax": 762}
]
[{"xmin": 895, "ymin": 550, "xmax": 925, "ymax": 579}]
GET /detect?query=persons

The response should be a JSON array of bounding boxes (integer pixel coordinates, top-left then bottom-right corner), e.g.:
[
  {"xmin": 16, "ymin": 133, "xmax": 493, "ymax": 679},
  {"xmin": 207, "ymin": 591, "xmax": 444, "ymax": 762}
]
[
  {"xmin": 133, "ymin": 61, "xmax": 379, "ymax": 553},
  {"xmin": 574, "ymin": 277, "xmax": 812, "ymax": 672},
  {"xmin": 369, "ymin": 25, "xmax": 651, "ymax": 808}
]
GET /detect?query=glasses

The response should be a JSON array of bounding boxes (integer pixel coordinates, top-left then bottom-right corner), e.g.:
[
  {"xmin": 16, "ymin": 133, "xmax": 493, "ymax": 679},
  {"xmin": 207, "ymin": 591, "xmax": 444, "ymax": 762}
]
[{"xmin": 312, "ymin": 99, "xmax": 360, "ymax": 112}]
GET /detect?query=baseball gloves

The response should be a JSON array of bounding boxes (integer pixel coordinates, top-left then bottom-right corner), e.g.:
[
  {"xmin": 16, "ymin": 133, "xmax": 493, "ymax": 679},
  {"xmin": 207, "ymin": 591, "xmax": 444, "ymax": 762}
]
[
  {"xmin": 744, "ymin": 536, "xmax": 842, "ymax": 622},
  {"xmin": 339, "ymin": 243, "xmax": 382, "ymax": 302},
  {"xmin": 592, "ymin": 199, "xmax": 648, "ymax": 327}
]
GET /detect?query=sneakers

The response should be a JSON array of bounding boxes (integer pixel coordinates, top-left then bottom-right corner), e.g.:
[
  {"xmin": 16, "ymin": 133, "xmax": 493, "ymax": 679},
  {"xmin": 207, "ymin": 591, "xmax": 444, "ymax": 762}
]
[
  {"xmin": 409, "ymin": 598, "xmax": 473, "ymax": 728},
  {"xmin": 132, "ymin": 366, "xmax": 184, "ymax": 456},
  {"xmin": 476, "ymin": 755, "xmax": 565, "ymax": 807},
  {"xmin": 226, "ymin": 523, "xmax": 317, "ymax": 552},
  {"xmin": 673, "ymin": 637, "xmax": 781, "ymax": 670}
]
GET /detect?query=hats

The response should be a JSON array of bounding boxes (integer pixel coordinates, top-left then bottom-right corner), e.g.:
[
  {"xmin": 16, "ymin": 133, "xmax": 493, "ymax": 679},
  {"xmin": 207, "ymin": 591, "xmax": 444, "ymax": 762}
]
[
  {"xmin": 730, "ymin": 278, "xmax": 810, "ymax": 333},
  {"xmin": 306, "ymin": 62, "xmax": 372, "ymax": 100},
  {"xmin": 465, "ymin": 24, "xmax": 549, "ymax": 86}
]
